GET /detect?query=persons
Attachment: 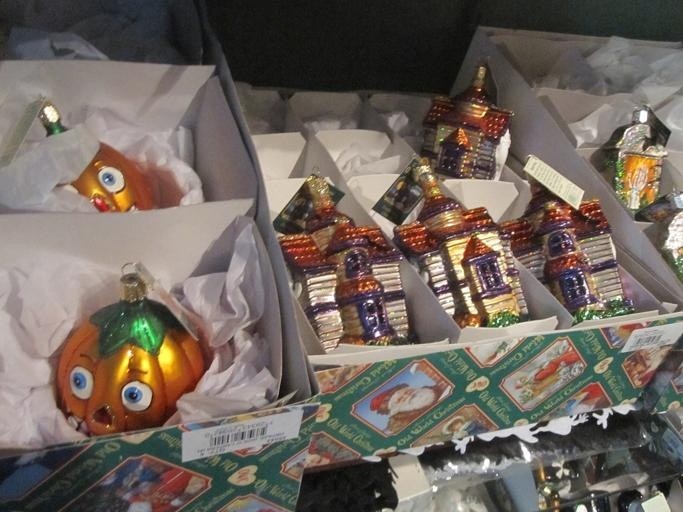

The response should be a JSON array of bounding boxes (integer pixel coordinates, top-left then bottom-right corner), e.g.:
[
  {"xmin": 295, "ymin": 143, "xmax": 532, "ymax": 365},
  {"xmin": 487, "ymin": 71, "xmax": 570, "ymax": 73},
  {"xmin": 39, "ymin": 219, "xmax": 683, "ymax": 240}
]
[
  {"xmin": 442, "ymin": 415, "xmax": 488, "ymax": 440},
  {"xmin": 516, "ymin": 351, "xmax": 579, "ymax": 390},
  {"xmin": 369, "ymin": 384, "xmax": 444, "ymax": 433}
]
